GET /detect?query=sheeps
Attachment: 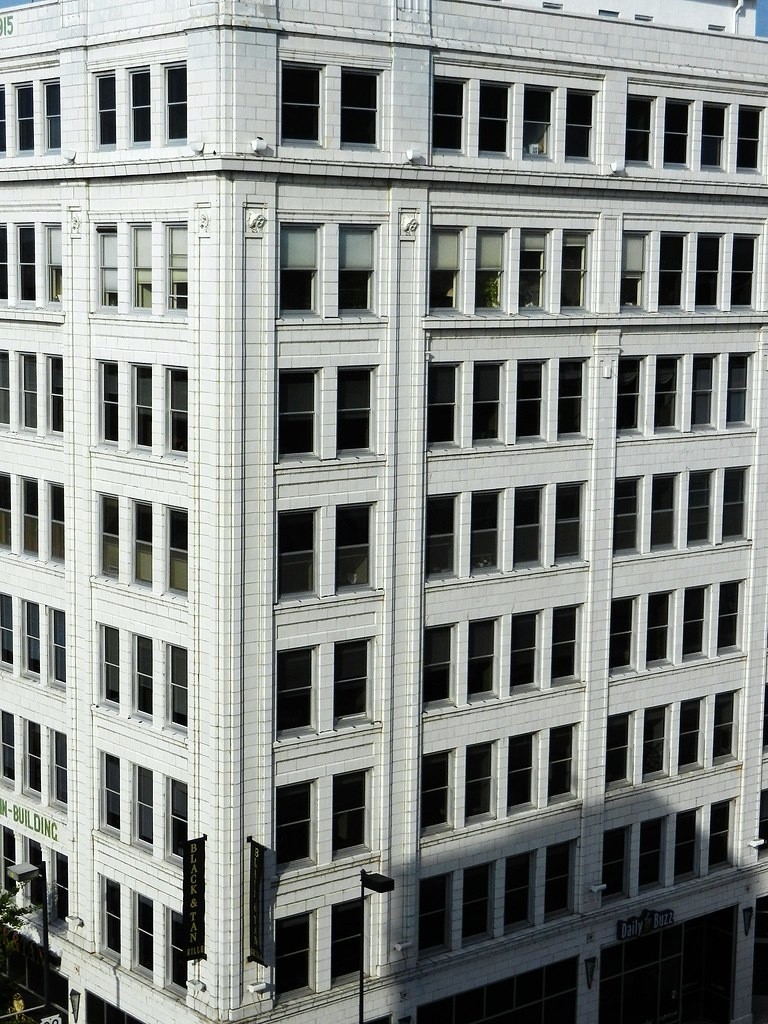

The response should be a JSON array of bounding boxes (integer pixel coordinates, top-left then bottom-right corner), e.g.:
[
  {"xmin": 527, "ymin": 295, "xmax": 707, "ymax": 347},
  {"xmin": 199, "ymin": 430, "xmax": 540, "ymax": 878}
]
[
  {"xmin": 400, "ymin": 215, "xmax": 418, "ymax": 232},
  {"xmin": 246, "ymin": 211, "xmax": 266, "ymax": 229}
]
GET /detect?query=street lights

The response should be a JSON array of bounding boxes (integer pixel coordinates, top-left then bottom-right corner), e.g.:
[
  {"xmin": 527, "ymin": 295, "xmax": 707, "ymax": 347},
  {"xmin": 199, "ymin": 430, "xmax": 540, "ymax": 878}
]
[
  {"xmin": 7, "ymin": 861, "xmax": 51, "ymax": 1017},
  {"xmin": 358, "ymin": 867, "xmax": 396, "ymax": 1024}
]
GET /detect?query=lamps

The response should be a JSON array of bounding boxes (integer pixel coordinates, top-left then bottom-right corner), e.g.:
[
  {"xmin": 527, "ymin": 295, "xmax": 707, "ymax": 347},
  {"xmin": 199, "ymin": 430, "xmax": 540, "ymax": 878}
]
[
  {"xmin": 748, "ymin": 839, "xmax": 764, "ymax": 847},
  {"xmin": 589, "ymin": 884, "xmax": 607, "ymax": 893},
  {"xmin": 249, "ymin": 981, "xmax": 266, "ymax": 993},
  {"xmin": 392, "ymin": 940, "xmax": 413, "ymax": 951},
  {"xmin": 65, "ymin": 915, "xmax": 83, "ymax": 925},
  {"xmin": 186, "ymin": 979, "xmax": 204, "ymax": 990}
]
